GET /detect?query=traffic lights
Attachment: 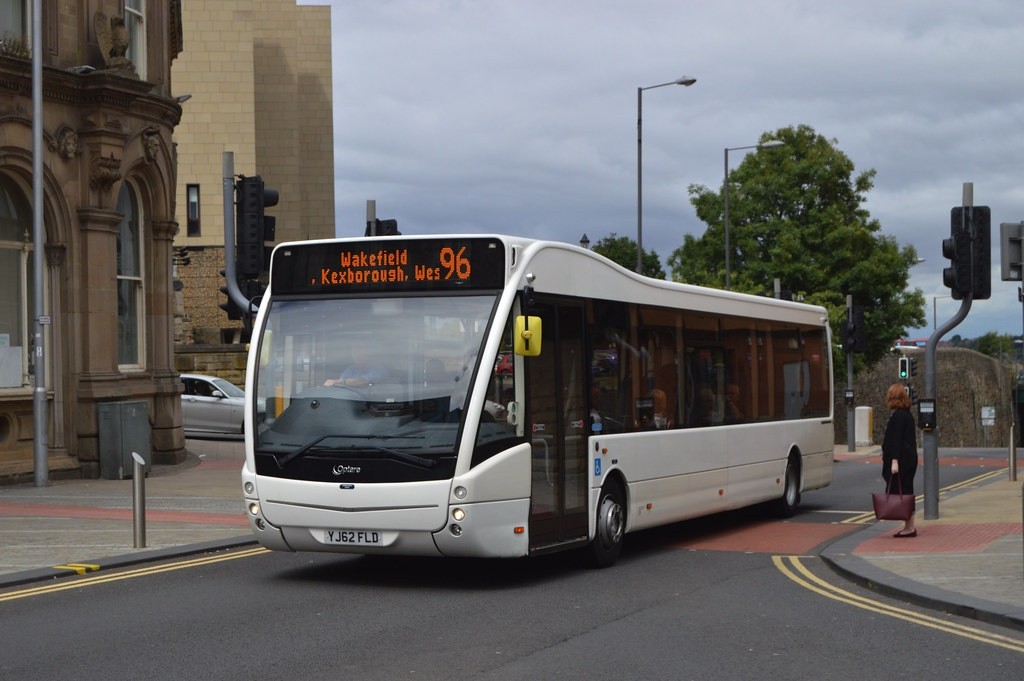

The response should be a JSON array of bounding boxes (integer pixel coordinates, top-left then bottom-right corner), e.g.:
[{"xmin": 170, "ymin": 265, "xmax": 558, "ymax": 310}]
[
  {"xmin": 943, "ymin": 232, "xmax": 972, "ymax": 294},
  {"xmin": 910, "ymin": 358, "xmax": 917, "ymax": 376},
  {"xmin": 899, "ymin": 358, "xmax": 909, "ymax": 379},
  {"xmin": 846, "ymin": 322, "xmax": 856, "ymax": 349},
  {"xmin": 236, "ymin": 174, "xmax": 279, "ymax": 275},
  {"xmin": 910, "ymin": 387, "xmax": 918, "ymax": 404}
]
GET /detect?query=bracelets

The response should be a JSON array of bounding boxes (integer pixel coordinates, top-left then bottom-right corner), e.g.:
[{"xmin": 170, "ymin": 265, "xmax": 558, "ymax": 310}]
[{"xmin": 340, "ymin": 378, "xmax": 345, "ymax": 385}]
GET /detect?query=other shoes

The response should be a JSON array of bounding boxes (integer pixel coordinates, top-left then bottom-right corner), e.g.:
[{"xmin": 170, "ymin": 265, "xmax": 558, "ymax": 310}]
[{"xmin": 893, "ymin": 530, "xmax": 916, "ymax": 537}]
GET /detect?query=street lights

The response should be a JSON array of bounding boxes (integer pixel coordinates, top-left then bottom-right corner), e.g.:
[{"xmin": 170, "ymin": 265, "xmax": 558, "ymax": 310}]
[
  {"xmin": 725, "ymin": 140, "xmax": 785, "ymax": 290},
  {"xmin": 219, "ymin": 269, "xmax": 244, "ymax": 320},
  {"xmin": 637, "ymin": 75, "xmax": 697, "ymax": 275}
]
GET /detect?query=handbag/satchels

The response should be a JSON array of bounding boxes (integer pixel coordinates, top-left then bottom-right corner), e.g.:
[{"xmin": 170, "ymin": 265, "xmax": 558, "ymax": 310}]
[{"xmin": 872, "ymin": 474, "xmax": 915, "ymax": 521}]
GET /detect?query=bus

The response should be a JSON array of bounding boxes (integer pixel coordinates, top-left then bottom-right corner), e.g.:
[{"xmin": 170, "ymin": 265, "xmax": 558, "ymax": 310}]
[{"xmin": 241, "ymin": 233, "xmax": 834, "ymax": 567}]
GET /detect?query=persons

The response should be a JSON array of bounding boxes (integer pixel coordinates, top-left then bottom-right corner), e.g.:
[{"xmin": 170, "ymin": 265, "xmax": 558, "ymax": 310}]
[
  {"xmin": 502, "ymin": 388, "xmax": 513, "ymax": 417},
  {"xmin": 688, "ymin": 388, "xmax": 714, "ymax": 426},
  {"xmin": 195, "ymin": 382, "xmax": 212, "ymax": 396},
  {"xmin": 882, "ymin": 383, "xmax": 919, "ymax": 538},
  {"xmin": 426, "ymin": 359, "xmax": 446, "ymax": 388},
  {"xmin": 323, "ymin": 341, "xmax": 388, "ymax": 402},
  {"xmin": 726, "ymin": 383, "xmax": 745, "ymax": 422},
  {"xmin": 647, "ymin": 389, "xmax": 668, "ymax": 428}
]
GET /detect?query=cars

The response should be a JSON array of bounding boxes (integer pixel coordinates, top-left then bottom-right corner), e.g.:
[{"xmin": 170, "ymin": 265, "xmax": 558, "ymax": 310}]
[{"xmin": 180, "ymin": 374, "xmax": 266, "ymax": 439}]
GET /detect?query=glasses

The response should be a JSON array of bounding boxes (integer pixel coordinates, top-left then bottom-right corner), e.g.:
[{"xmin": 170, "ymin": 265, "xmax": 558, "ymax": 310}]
[{"xmin": 725, "ymin": 392, "xmax": 736, "ymax": 395}]
